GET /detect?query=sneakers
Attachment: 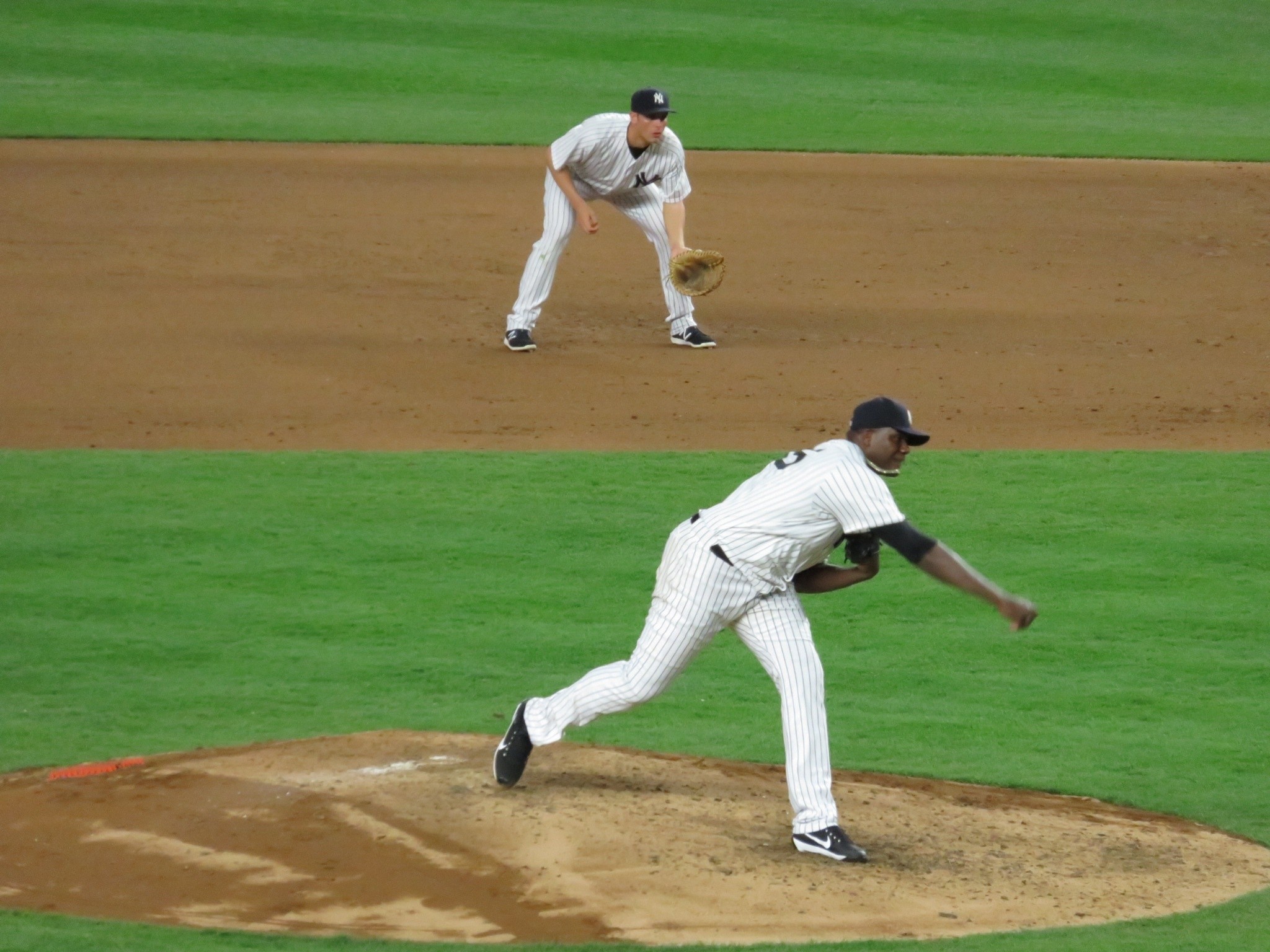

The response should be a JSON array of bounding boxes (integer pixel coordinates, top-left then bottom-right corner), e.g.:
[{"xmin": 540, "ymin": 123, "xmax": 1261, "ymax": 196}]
[
  {"xmin": 791, "ymin": 822, "xmax": 869, "ymax": 863},
  {"xmin": 670, "ymin": 324, "xmax": 716, "ymax": 349},
  {"xmin": 503, "ymin": 328, "xmax": 538, "ymax": 351},
  {"xmin": 493, "ymin": 698, "xmax": 536, "ymax": 788}
]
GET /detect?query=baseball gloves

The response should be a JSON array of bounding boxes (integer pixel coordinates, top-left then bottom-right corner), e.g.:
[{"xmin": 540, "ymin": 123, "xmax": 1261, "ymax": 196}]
[
  {"xmin": 843, "ymin": 533, "xmax": 884, "ymax": 565},
  {"xmin": 663, "ymin": 248, "xmax": 726, "ymax": 300}
]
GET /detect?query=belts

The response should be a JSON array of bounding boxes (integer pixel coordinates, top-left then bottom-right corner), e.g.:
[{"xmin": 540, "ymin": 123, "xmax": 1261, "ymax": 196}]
[{"xmin": 690, "ymin": 512, "xmax": 734, "ymax": 565}]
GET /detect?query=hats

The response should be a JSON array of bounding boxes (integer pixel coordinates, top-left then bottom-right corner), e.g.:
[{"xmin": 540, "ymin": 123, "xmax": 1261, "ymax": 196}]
[
  {"xmin": 630, "ymin": 90, "xmax": 677, "ymax": 116},
  {"xmin": 849, "ymin": 395, "xmax": 930, "ymax": 448}
]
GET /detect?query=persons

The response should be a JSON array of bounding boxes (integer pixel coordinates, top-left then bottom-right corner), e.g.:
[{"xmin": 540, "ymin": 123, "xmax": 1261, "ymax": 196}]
[
  {"xmin": 503, "ymin": 89, "xmax": 717, "ymax": 350},
  {"xmin": 492, "ymin": 398, "xmax": 1037, "ymax": 863}
]
